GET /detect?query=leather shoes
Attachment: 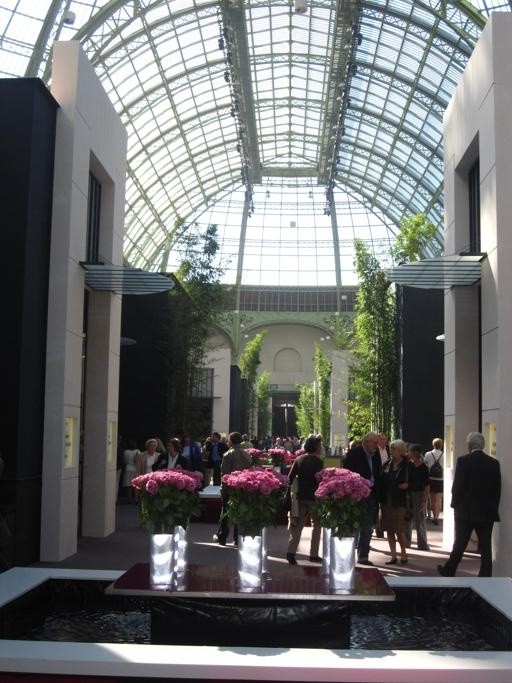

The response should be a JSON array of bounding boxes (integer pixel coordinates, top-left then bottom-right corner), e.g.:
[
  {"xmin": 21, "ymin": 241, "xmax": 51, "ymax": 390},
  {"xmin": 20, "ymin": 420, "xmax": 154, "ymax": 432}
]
[
  {"xmin": 400, "ymin": 558, "xmax": 408, "ymax": 563},
  {"xmin": 286, "ymin": 552, "xmax": 296, "ymax": 564},
  {"xmin": 213, "ymin": 533, "xmax": 226, "ymax": 545},
  {"xmin": 385, "ymin": 558, "xmax": 397, "ymax": 564},
  {"xmin": 309, "ymin": 555, "xmax": 323, "ymax": 562},
  {"xmin": 358, "ymin": 560, "xmax": 373, "ymax": 565},
  {"xmin": 438, "ymin": 564, "xmax": 445, "ymax": 575}
]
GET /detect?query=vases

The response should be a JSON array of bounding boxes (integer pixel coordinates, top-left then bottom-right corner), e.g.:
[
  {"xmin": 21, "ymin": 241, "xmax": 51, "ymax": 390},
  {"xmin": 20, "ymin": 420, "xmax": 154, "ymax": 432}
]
[
  {"xmin": 321, "ymin": 525, "xmax": 360, "ymax": 594},
  {"xmin": 236, "ymin": 528, "xmax": 271, "ymax": 593},
  {"xmin": 150, "ymin": 527, "xmax": 189, "ymax": 591}
]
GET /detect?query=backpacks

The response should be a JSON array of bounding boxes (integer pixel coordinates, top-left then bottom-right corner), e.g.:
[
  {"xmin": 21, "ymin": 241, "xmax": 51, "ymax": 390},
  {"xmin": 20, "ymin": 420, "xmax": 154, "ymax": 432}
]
[{"xmin": 429, "ymin": 451, "xmax": 443, "ymax": 478}]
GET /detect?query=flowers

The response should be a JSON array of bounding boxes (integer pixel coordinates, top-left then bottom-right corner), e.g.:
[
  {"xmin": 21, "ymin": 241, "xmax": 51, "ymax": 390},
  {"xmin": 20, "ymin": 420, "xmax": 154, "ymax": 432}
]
[
  {"xmin": 216, "ymin": 467, "xmax": 290, "ymax": 537},
  {"xmin": 131, "ymin": 472, "xmax": 204, "ymax": 534},
  {"xmin": 306, "ymin": 467, "xmax": 380, "ymax": 539},
  {"xmin": 244, "ymin": 447, "xmax": 286, "ymax": 465}
]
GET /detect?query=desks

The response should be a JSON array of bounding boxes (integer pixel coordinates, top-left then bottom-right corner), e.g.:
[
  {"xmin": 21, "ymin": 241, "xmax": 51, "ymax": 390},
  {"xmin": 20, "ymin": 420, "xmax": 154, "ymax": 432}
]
[{"xmin": 103, "ymin": 562, "xmax": 398, "ymax": 648}]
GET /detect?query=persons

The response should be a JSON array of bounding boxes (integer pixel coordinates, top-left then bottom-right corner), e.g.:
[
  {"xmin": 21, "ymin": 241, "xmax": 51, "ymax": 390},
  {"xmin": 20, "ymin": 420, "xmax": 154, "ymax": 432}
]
[
  {"xmin": 285, "ymin": 434, "xmax": 325, "ymax": 565},
  {"xmin": 115, "ymin": 429, "xmax": 448, "ymax": 527},
  {"xmin": 402, "ymin": 445, "xmax": 429, "ymax": 552},
  {"xmin": 343, "ymin": 433, "xmax": 381, "ymax": 564},
  {"xmin": 437, "ymin": 432, "xmax": 502, "ymax": 577},
  {"xmin": 212, "ymin": 431, "xmax": 252, "ymax": 547},
  {"xmin": 377, "ymin": 439, "xmax": 418, "ymax": 567}
]
